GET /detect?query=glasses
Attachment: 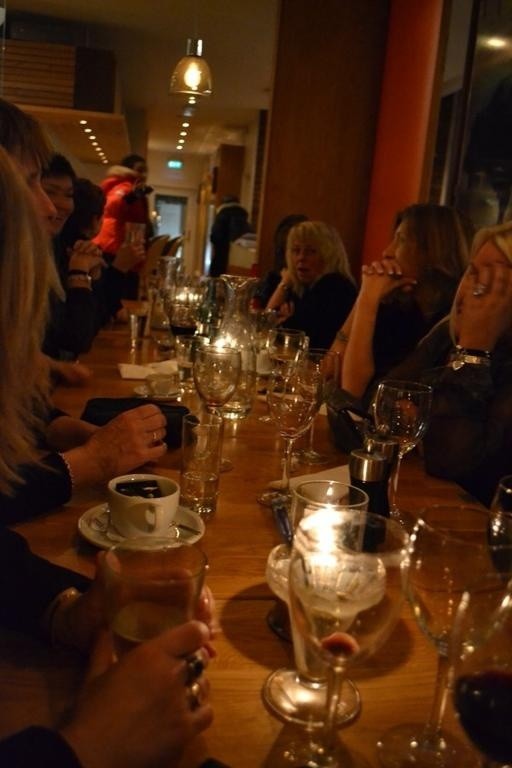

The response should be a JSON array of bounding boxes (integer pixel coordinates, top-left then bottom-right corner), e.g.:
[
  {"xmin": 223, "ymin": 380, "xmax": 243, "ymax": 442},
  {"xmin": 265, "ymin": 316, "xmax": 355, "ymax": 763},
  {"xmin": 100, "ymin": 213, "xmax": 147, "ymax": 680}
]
[{"xmin": 162, "ymin": 1, "xmax": 213, "ymax": 98}]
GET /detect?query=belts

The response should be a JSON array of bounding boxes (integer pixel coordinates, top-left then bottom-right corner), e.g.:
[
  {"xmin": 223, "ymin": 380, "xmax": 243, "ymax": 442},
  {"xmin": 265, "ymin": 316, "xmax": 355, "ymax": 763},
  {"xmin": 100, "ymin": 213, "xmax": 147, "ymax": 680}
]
[
  {"xmin": 272, "ymin": 509, "xmax": 412, "ymax": 768},
  {"xmin": 187, "ymin": 345, "xmax": 241, "ymax": 472},
  {"xmin": 264, "ymin": 476, "xmax": 367, "ymax": 730},
  {"xmin": 379, "ymin": 506, "xmax": 512, "ymax": 767},
  {"xmin": 372, "ymin": 378, "xmax": 436, "ymax": 526},
  {"xmin": 485, "ymin": 477, "xmax": 512, "ymax": 607},
  {"xmin": 168, "ymin": 304, "xmax": 198, "ymax": 386},
  {"xmin": 259, "ymin": 358, "xmax": 320, "ymax": 506},
  {"xmin": 257, "ymin": 330, "xmax": 305, "ymax": 426},
  {"xmin": 292, "ymin": 352, "xmax": 339, "ymax": 466}
]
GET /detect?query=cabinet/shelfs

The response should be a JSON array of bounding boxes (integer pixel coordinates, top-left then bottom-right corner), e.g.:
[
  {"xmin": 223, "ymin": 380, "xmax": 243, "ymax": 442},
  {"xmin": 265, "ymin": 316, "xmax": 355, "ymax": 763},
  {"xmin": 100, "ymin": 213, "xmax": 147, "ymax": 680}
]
[{"xmin": 349, "ymin": 446, "xmax": 389, "ymax": 548}]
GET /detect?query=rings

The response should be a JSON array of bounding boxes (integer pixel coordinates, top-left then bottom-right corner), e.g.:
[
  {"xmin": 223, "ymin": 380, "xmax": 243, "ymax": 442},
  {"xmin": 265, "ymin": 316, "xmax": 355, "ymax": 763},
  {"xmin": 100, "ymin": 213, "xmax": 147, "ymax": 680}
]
[
  {"xmin": 473, "ymin": 284, "xmax": 486, "ymax": 295},
  {"xmin": 152, "ymin": 432, "xmax": 157, "ymax": 440},
  {"xmin": 186, "ymin": 681, "xmax": 206, "ymax": 710},
  {"xmin": 184, "ymin": 652, "xmax": 206, "ymax": 678}
]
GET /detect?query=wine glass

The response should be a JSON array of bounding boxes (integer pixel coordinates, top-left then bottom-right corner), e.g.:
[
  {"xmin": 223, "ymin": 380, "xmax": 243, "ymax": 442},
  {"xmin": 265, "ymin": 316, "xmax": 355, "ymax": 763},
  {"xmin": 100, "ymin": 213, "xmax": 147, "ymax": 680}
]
[
  {"xmin": 186, "ymin": 681, "xmax": 206, "ymax": 710},
  {"xmin": 184, "ymin": 652, "xmax": 206, "ymax": 678}
]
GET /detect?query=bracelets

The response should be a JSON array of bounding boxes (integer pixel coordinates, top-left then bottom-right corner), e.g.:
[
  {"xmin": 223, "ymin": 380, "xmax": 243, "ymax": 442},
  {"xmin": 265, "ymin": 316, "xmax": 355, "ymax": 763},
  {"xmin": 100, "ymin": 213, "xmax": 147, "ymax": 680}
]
[
  {"xmin": 455, "ymin": 344, "xmax": 491, "ymax": 370},
  {"xmin": 279, "ymin": 280, "xmax": 291, "ymax": 294},
  {"xmin": 59, "ymin": 451, "xmax": 75, "ymax": 489},
  {"xmin": 69, "ymin": 269, "xmax": 88, "ymax": 277}
]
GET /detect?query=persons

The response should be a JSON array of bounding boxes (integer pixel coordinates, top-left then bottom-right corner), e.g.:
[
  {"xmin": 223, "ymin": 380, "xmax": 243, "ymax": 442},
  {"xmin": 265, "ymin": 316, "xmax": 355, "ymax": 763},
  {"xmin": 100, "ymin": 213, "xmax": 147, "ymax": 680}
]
[
  {"xmin": 88, "ymin": 154, "xmax": 154, "ymax": 266},
  {"xmin": 254, "ymin": 213, "xmax": 309, "ymax": 310},
  {"xmin": 0, "ymin": 97, "xmax": 58, "ymax": 235},
  {"xmin": 34, "ymin": 154, "xmax": 125, "ymax": 366},
  {"xmin": 41, "ymin": 357, "xmax": 90, "ymax": 384},
  {"xmin": 53, "ymin": 177, "xmax": 145, "ymax": 325},
  {"xmin": 0, "ymin": 147, "xmax": 182, "ymax": 524},
  {"xmin": 363, "ymin": 220, "xmax": 511, "ymax": 512},
  {"xmin": 1, "ymin": 518, "xmax": 219, "ymax": 768},
  {"xmin": 208, "ymin": 191, "xmax": 252, "ymax": 279},
  {"xmin": 318, "ymin": 204, "xmax": 461, "ymax": 453},
  {"xmin": 263, "ymin": 219, "xmax": 358, "ymax": 367}
]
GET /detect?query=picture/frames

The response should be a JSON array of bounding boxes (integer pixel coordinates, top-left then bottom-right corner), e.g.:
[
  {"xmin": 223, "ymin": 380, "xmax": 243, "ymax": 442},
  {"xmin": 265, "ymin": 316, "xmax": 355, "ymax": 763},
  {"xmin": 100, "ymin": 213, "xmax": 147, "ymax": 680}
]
[{"xmin": 0, "ymin": 327, "xmax": 512, "ymax": 768}]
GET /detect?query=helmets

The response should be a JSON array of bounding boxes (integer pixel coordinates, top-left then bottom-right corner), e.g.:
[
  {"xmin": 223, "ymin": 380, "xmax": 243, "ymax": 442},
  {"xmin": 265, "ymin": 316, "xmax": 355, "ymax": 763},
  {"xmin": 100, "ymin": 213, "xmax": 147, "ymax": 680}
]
[
  {"xmin": 133, "ymin": 382, "xmax": 188, "ymax": 403},
  {"xmin": 77, "ymin": 503, "xmax": 208, "ymax": 552}
]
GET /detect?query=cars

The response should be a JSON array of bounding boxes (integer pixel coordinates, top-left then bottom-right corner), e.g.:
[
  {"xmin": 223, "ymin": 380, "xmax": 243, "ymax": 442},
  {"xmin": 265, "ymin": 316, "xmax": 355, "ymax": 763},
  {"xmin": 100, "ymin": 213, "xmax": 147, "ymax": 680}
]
[
  {"xmin": 151, "ymin": 253, "xmax": 185, "ymax": 350},
  {"xmin": 106, "ymin": 472, "xmax": 182, "ymax": 540},
  {"xmin": 213, "ymin": 273, "xmax": 258, "ymax": 389},
  {"xmin": 104, "ymin": 537, "xmax": 210, "ymax": 668},
  {"xmin": 178, "ymin": 413, "xmax": 224, "ymax": 517}
]
